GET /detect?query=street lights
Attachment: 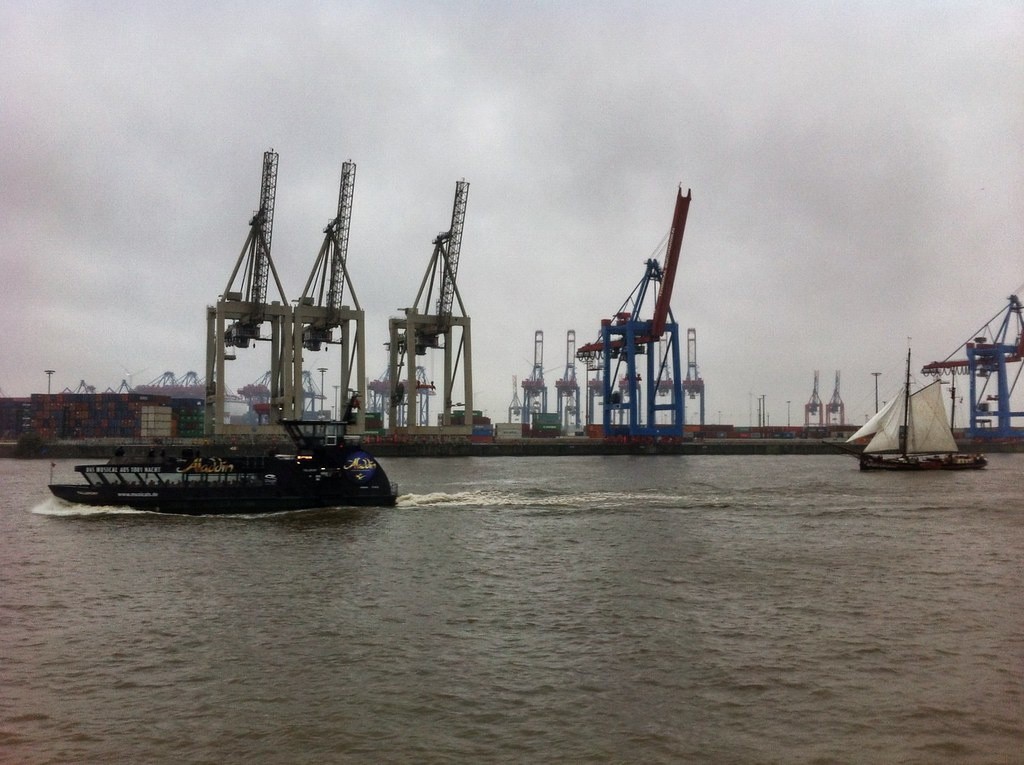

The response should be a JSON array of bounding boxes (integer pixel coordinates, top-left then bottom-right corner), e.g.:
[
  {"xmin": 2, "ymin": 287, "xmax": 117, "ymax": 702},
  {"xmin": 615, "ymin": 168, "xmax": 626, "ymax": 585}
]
[
  {"xmin": 44, "ymin": 368, "xmax": 54, "ymax": 395},
  {"xmin": 786, "ymin": 400, "xmax": 792, "ymax": 428},
  {"xmin": 871, "ymin": 372, "xmax": 882, "ymax": 416},
  {"xmin": 760, "ymin": 396, "xmax": 772, "ymax": 438}
]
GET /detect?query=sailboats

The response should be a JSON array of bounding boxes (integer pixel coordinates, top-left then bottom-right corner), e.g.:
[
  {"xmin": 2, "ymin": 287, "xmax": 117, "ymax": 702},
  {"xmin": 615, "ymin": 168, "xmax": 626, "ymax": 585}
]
[{"xmin": 821, "ymin": 348, "xmax": 989, "ymax": 473}]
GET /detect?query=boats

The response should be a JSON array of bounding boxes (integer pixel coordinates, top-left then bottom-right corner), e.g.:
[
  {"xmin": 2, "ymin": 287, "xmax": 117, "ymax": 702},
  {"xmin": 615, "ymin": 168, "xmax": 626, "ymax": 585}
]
[{"xmin": 49, "ymin": 442, "xmax": 399, "ymax": 516}]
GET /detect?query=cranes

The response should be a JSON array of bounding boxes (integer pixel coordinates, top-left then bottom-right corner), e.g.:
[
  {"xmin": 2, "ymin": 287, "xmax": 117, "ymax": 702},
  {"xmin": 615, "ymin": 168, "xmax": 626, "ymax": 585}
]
[
  {"xmin": 805, "ymin": 371, "xmax": 843, "ymax": 432},
  {"xmin": 494, "ymin": 184, "xmax": 704, "ymax": 449},
  {"xmin": 201, "ymin": 146, "xmax": 494, "ymax": 448}
]
